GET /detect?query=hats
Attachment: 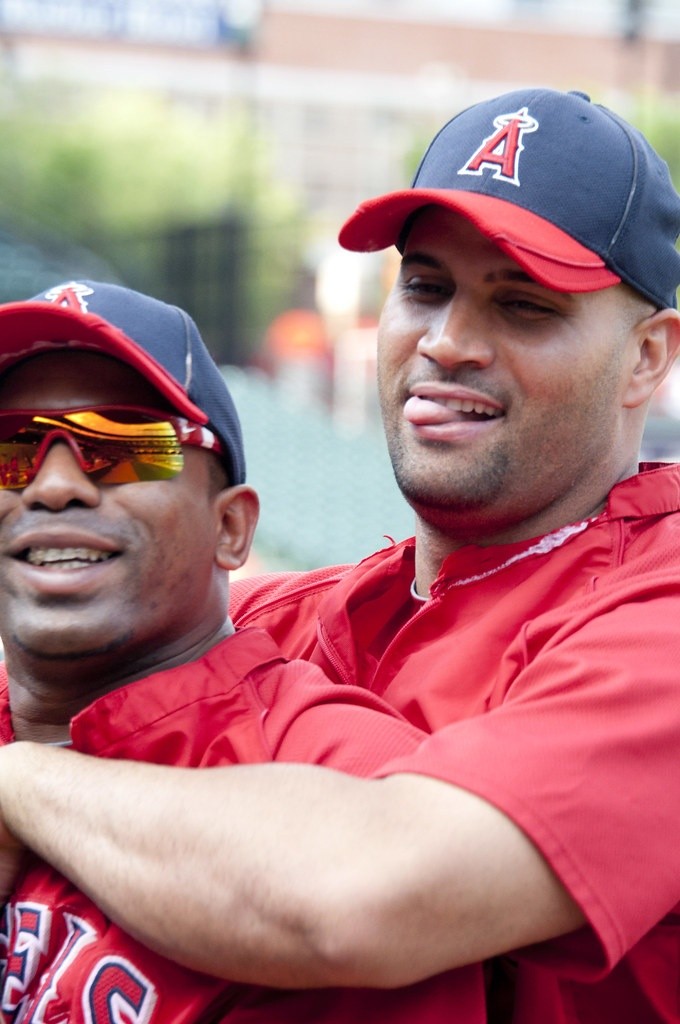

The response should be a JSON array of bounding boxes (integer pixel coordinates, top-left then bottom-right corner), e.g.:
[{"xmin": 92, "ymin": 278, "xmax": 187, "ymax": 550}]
[
  {"xmin": 0, "ymin": 280, "xmax": 246, "ymax": 486},
  {"xmin": 338, "ymin": 89, "xmax": 680, "ymax": 309}
]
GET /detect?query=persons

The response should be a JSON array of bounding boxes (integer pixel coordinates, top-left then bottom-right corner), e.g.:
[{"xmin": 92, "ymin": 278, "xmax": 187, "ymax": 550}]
[
  {"xmin": 0, "ymin": 278, "xmax": 486, "ymax": 1024},
  {"xmin": 0, "ymin": 86, "xmax": 680, "ymax": 1024},
  {"xmin": 0, "ymin": 453, "xmax": 35, "ymax": 486}
]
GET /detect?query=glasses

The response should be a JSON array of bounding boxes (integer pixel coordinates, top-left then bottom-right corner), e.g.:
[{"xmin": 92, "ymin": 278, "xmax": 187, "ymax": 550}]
[{"xmin": 0, "ymin": 405, "xmax": 224, "ymax": 490}]
